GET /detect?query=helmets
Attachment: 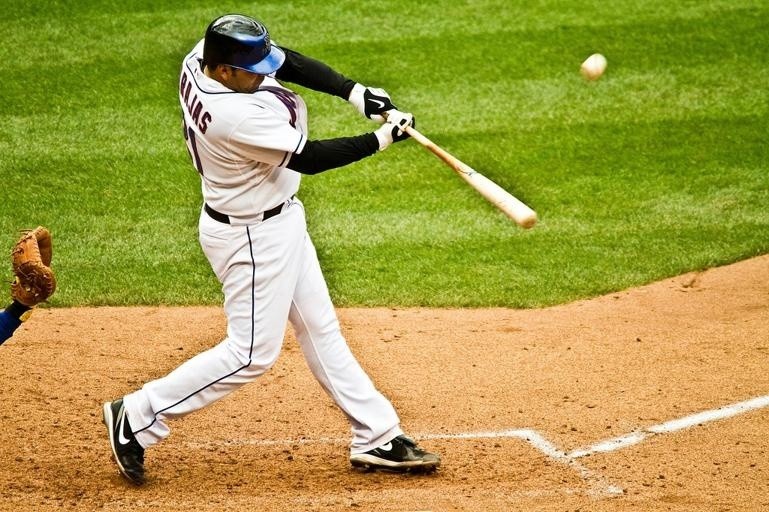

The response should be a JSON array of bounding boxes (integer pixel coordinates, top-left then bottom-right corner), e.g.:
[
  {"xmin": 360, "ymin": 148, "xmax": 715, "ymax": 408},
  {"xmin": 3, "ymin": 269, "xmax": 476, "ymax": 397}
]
[{"xmin": 203, "ymin": 12, "xmax": 287, "ymax": 76}]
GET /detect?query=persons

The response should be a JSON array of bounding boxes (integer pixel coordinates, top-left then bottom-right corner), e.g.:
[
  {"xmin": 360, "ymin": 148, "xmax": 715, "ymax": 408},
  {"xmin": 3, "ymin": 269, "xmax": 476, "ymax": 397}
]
[
  {"xmin": 102, "ymin": 13, "xmax": 440, "ymax": 487},
  {"xmin": 0, "ymin": 226, "xmax": 56, "ymax": 346}
]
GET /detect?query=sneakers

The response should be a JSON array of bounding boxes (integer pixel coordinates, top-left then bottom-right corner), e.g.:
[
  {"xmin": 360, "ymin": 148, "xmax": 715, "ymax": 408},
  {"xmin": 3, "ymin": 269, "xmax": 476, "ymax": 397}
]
[
  {"xmin": 101, "ymin": 399, "xmax": 147, "ymax": 487},
  {"xmin": 350, "ymin": 433, "xmax": 442, "ymax": 474}
]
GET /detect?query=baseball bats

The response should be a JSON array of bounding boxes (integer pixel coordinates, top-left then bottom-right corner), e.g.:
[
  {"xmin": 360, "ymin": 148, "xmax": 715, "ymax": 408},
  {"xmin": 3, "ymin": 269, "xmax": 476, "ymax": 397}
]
[{"xmin": 385, "ymin": 112, "xmax": 537, "ymax": 228}]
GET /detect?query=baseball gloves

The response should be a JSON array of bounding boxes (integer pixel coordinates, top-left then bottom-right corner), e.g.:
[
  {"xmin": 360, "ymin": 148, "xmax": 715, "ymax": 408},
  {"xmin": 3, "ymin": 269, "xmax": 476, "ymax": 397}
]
[{"xmin": 12, "ymin": 226, "xmax": 57, "ymax": 306}]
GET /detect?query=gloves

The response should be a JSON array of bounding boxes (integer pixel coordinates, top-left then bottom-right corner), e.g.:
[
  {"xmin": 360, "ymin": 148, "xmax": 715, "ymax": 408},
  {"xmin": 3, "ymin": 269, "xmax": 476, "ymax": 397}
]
[
  {"xmin": 373, "ymin": 110, "xmax": 415, "ymax": 154},
  {"xmin": 348, "ymin": 79, "xmax": 399, "ymax": 125}
]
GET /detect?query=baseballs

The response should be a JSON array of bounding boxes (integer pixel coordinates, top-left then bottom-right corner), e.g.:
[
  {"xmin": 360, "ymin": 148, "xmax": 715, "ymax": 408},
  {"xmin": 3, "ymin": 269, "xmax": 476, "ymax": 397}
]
[{"xmin": 581, "ymin": 53, "xmax": 606, "ymax": 80}]
